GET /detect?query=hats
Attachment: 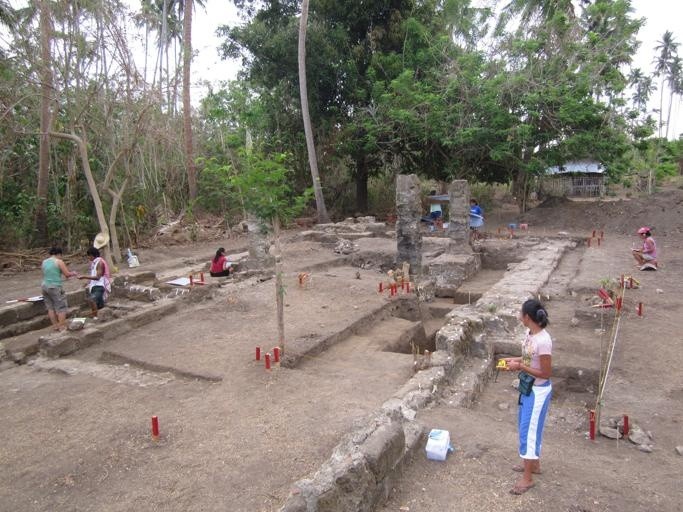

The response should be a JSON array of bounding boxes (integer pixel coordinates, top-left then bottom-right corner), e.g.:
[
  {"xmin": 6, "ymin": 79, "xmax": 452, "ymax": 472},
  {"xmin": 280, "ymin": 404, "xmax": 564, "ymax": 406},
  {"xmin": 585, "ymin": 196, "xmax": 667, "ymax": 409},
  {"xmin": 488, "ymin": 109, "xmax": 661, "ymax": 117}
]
[
  {"xmin": 636, "ymin": 227, "xmax": 650, "ymax": 234},
  {"xmin": 93, "ymin": 232, "xmax": 110, "ymax": 250}
]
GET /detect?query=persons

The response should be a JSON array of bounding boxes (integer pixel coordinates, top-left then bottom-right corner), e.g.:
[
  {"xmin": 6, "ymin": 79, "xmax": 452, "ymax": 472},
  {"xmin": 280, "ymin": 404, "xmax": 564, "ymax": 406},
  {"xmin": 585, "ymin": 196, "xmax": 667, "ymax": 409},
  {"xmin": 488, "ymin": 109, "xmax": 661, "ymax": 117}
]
[
  {"xmin": 421, "ymin": 191, "xmax": 441, "ymax": 226},
  {"xmin": 631, "ymin": 227, "xmax": 656, "ymax": 269},
  {"xmin": 470, "ymin": 199, "xmax": 483, "ymax": 231},
  {"xmin": 78, "ymin": 249, "xmax": 111, "ymax": 310},
  {"xmin": 209, "ymin": 248, "xmax": 233, "ymax": 277},
  {"xmin": 497, "ymin": 298, "xmax": 552, "ymax": 497},
  {"xmin": 40, "ymin": 247, "xmax": 77, "ymax": 331}
]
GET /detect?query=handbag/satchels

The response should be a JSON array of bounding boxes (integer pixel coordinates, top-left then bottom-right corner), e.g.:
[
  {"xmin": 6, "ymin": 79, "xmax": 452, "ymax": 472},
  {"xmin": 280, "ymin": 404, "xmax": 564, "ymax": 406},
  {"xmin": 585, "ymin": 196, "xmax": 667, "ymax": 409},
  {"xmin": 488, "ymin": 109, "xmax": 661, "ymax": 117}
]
[{"xmin": 517, "ymin": 371, "xmax": 535, "ymax": 396}]
